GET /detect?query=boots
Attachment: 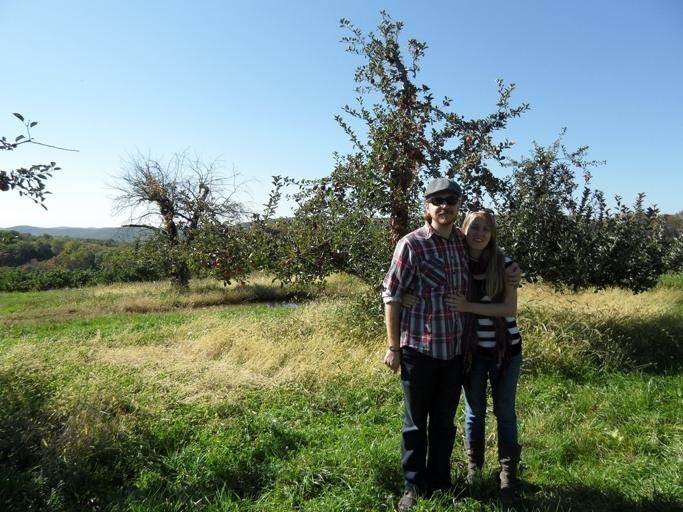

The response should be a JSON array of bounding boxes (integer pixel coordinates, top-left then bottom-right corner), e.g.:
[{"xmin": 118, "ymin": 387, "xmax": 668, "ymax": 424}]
[
  {"xmin": 460, "ymin": 448, "xmax": 485, "ymax": 486},
  {"xmin": 498, "ymin": 457, "xmax": 518, "ymax": 493}
]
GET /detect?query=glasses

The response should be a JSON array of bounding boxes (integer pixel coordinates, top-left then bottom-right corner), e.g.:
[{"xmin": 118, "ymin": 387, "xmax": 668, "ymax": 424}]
[{"xmin": 427, "ymin": 196, "xmax": 460, "ymax": 206}]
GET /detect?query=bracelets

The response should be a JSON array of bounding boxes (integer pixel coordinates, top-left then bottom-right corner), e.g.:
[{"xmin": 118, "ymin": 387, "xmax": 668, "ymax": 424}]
[{"xmin": 388, "ymin": 346, "xmax": 400, "ymax": 352}]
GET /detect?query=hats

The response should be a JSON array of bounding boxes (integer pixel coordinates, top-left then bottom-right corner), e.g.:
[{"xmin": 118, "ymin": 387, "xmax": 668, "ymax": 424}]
[{"xmin": 422, "ymin": 177, "xmax": 461, "ymax": 196}]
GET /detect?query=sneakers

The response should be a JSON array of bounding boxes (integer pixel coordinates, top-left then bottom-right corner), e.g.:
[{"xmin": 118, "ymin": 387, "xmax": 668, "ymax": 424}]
[{"xmin": 394, "ymin": 477, "xmax": 420, "ymax": 512}]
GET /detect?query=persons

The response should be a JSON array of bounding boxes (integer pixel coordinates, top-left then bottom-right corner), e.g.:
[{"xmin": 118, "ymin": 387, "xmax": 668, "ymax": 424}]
[
  {"xmin": 377, "ymin": 176, "xmax": 522, "ymax": 509},
  {"xmin": 402, "ymin": 203, "xmax": 528, "ymax": 494}
]
